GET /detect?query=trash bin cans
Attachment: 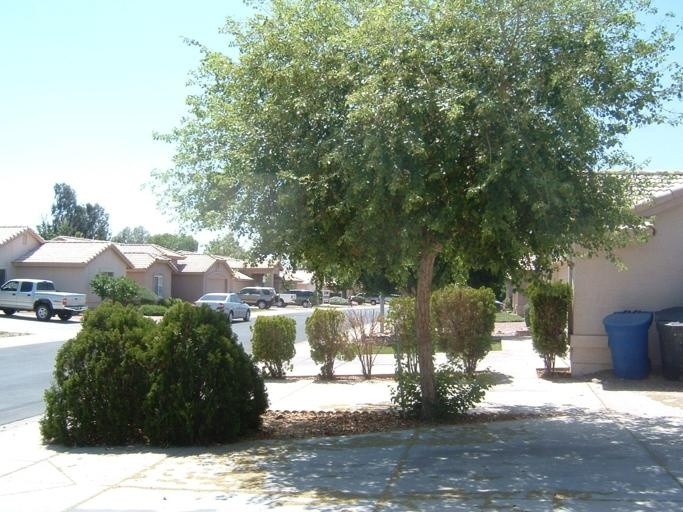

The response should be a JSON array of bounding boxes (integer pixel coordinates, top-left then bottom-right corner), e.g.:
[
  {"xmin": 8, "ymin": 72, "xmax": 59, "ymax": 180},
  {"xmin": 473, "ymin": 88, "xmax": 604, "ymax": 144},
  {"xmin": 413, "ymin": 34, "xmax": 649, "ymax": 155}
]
[{"xmin": 603, "ymin": 306, "xmax": 683, "ymax": 380}]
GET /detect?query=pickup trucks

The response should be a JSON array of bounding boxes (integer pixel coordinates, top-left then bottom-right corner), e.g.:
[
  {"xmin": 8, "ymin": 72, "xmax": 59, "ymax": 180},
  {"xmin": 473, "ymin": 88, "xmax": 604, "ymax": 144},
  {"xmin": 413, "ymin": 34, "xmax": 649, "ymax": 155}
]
[{"xmin": 0, "ymin": 278, "xmax": 88, "ymax": 321}]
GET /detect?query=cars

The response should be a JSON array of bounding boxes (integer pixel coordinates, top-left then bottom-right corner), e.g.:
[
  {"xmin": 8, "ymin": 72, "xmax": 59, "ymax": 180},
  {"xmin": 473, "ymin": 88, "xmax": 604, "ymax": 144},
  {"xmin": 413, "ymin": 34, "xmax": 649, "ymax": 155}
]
[
  {"xmin": 351, "ymin": 293, "xmax": 380, "ymax": 305},
  {"xmin": 193, "ymin": 292, "xmax": 250, "ymax": 323}
]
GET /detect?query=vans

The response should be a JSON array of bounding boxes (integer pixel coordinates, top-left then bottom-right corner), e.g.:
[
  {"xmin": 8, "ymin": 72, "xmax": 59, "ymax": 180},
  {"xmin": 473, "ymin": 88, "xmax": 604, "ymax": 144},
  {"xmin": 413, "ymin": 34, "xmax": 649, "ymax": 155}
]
[{"xmin": 237, "ymin": 286, "xmax": 314, "ymax": 309}]
